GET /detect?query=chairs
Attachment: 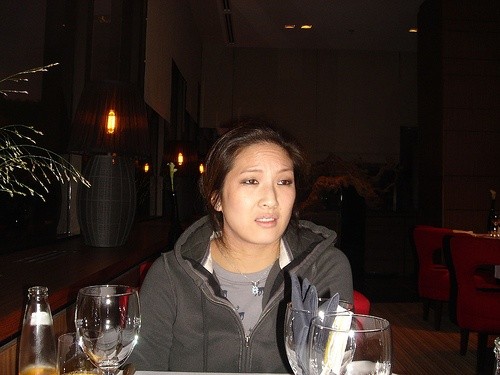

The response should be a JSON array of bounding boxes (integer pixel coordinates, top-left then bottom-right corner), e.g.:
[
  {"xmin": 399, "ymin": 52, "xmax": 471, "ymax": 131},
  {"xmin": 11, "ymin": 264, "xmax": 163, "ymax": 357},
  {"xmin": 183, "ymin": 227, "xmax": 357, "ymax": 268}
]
[
  {"xmin": 170, "ymin": 175, "xmax": 193, "ymax": 247},
  {"xmin": 444, "ymin": 232, "xmax": 500, "ymax": 375},
  {"xmin": 412, "ymin": 223, "xmax": 473, "ymax": 330}
]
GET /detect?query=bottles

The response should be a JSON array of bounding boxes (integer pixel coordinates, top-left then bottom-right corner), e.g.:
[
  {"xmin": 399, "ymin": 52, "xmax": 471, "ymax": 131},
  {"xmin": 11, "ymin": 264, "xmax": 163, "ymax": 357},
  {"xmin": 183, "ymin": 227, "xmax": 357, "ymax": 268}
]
[
  {"xmin": 486, "ymin": 199, "xmax": 496, "ymax": 233},
  {"xmin": 18, "ymin": 286, "xmax": 59, "ymax": 375}
]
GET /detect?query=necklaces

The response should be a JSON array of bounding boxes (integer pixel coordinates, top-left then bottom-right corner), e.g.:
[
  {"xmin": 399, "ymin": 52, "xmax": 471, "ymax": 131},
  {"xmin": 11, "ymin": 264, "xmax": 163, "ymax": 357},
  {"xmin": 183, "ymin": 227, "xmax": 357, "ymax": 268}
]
[{"xmin": 229, "ymin": 255, "xmax": 279, "ymax": 294}]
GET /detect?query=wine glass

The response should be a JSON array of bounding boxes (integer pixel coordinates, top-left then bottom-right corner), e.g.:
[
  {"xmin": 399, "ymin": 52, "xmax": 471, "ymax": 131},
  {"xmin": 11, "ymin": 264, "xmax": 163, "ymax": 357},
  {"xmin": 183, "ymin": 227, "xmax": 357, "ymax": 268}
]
[{"xmin": 74, "ymin": 285, "xmax": 141, "ymax": 375}]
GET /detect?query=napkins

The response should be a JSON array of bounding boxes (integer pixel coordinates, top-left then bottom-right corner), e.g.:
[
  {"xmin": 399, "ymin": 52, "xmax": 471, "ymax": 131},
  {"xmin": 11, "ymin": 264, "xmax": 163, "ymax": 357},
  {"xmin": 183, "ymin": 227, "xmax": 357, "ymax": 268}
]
[{"xmin": 290, "ymin": 270, "xmax": 340, "ymax": 375}]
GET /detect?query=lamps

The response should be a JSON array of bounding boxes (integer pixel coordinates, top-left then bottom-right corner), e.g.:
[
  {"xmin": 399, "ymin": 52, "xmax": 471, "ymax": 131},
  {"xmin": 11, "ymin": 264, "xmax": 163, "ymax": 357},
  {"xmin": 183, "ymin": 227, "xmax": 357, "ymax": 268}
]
[{"xmin": 67, "ymin": 78, "xmax": 150, "ymax": 248}]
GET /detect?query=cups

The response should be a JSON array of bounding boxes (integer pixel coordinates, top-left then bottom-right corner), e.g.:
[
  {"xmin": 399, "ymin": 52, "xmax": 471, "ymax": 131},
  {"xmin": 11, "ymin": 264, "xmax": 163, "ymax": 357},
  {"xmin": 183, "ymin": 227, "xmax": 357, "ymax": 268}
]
[
  {"xmin": 283, "ymin": 298, "xmax": 358, "ymax": 375},
  {"xmin": 58, "ymin": 331, "xmax": 104, "ymax": 375},
  {"xmin": 308, "ymin": 313, "xmax": 392, "ymax": 375},
  {"xmin": 488, "ymin": 209, "xmax": 500, "ymax": 238}
]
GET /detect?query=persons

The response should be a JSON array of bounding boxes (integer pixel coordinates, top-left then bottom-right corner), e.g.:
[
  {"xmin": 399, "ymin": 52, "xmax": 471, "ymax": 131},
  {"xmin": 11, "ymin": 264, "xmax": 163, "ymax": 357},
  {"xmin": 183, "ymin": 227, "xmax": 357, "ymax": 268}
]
[
  {"xmin": 121, "ymin": 127, "xmax": 354, "ymax": 375},
  {"xmin": 301, "ymin": 170, "xmax": 378, "ymax": 288}
]
